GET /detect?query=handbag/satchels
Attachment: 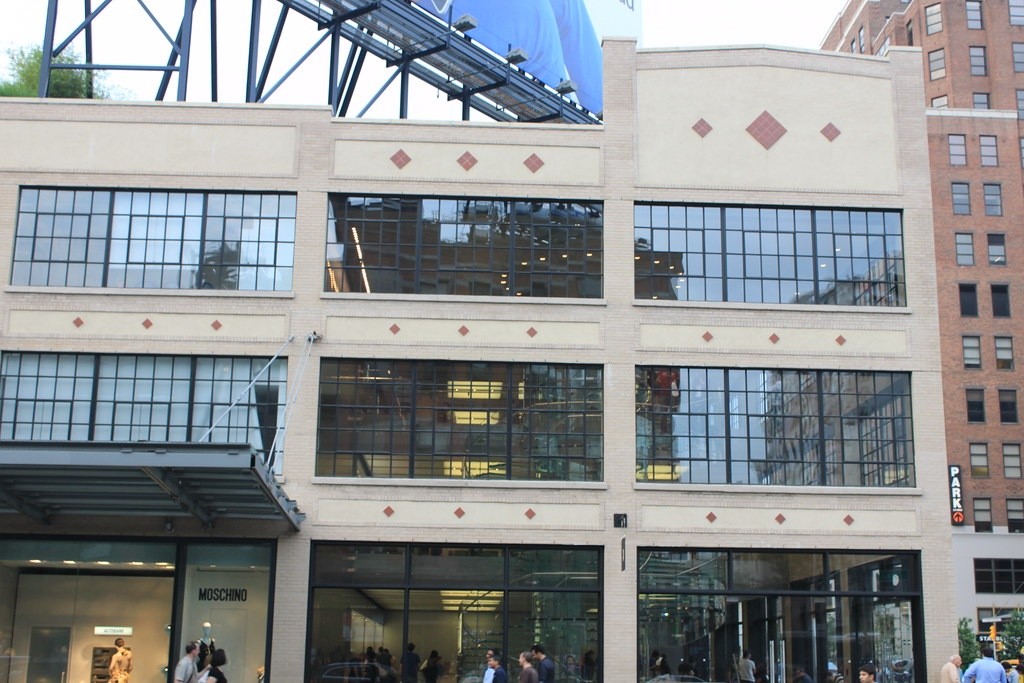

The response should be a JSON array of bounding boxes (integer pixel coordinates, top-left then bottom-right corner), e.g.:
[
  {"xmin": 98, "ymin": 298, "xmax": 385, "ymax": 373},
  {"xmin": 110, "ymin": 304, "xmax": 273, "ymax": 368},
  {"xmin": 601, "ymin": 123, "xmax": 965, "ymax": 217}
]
[{"xmin": 419, "ymin": 659, "xmax": 428, "ymax": 670}]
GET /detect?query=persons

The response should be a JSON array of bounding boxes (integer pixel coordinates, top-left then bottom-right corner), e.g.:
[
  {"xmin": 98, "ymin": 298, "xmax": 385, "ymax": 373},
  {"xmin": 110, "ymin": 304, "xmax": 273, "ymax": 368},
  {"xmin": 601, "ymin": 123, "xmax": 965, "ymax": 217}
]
[
  {"xmin": 483, "ymin": 648, "xmax": 507, "ymax": 683},
  {"xmin": 650, "ymin": 650, "xmax": 708, "ymax": 683},
  {"xmin": 561, "ymin": 650, "xmax": 598, "ymax": 683},
  {"xmin": 518, "ymin": 645, "xmax": 554, "ymax": 683},
  {"xmin": 423, "ymin": 650, "xmax": 439, "ymax": 683},
  {"xmin": 174, "ymin": 641, "xmax": 212, "ymax": 683},
  {"xmin": 362, "ymin": 647, "xmax": 396, "ymax": 678},
  {"xmin": 791, "ymin": 658, "xmax": 888, "ymax": 683},
  {"xmin": 400, "ymin": 643, "xmax": 421, "ymax": 683},
  {"xmin": 257, "ymin": 665, "xmax": 264, "ymax": 683},
  {"xmin": 205, "ymin": 649, "xmax": 228, "ymax": 683},
  {"xmin": 109, "ymin": 638, "xmax": 134, "ymax": 683},
  {"xmin": 940, "ymin": 647, "xmax": 1024, "ymax": 683},
  {"xmin": 195, "ymin": 622, "xmax": 216, "ymax": 673},
  {"xmin": 728, "ymin": 650, "xmax": 778, "ymax": 683}
]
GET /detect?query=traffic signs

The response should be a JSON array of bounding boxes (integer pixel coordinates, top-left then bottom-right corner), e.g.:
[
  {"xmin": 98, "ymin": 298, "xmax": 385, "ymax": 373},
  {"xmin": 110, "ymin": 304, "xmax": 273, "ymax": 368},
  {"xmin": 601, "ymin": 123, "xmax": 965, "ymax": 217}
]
[{"xmin": 976, "ymin": 633, "xmax": 1005, "ymax": 643}]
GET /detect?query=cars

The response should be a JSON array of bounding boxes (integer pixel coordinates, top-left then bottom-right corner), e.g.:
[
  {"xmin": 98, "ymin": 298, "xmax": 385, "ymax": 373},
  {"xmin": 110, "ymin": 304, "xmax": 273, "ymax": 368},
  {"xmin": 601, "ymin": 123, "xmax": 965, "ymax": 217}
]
[
  {"xmin": 645, "ymin": 674, "xmax": 706, "ymax": 683},
  {"xmin": 309, "ymin": 662, "xmax": 400, "ymax": 683},
  {"xmin": 1000, "ymin": 659, "xmax": 1024, "ymax": 683}
]
